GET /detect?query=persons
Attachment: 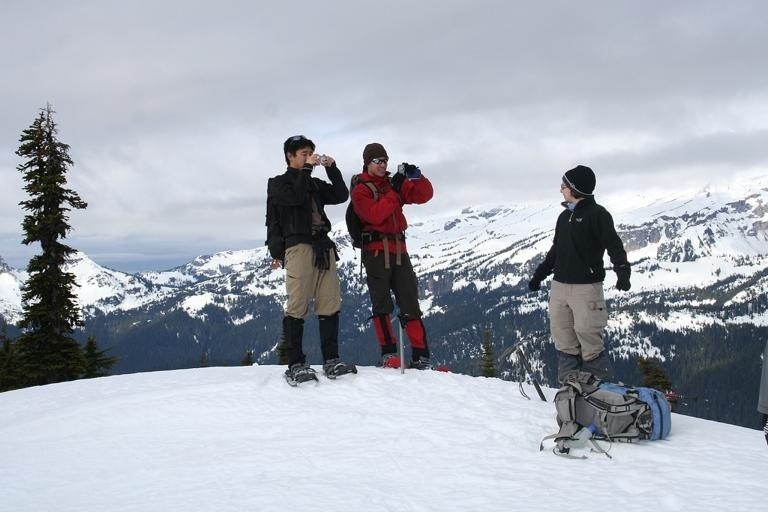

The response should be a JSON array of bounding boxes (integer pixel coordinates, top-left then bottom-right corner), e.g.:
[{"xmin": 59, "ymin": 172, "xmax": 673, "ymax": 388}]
[
  {"xmin": 265, "ymin": 134, "xmax": 359, "ymax": 383},
  {"xmin": 528, "ymin": 164, "xmax": 632, "ymax": 385},
  {"xmin": 345, "ymin": 142, "xmax": 435, "ymax": 370}
]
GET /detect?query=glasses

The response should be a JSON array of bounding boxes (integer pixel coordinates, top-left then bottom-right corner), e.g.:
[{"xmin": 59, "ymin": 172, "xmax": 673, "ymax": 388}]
[
  {"xmin": 371, "ymin": 159, "xmax": 389, "ymax": 163},
  {"xmin": 561, "ymin": 184, "xmax": 570, "ymax": 189},
  {"xmin": 289, "ymin": 135, "xmax": 306, "ymax": 148}
]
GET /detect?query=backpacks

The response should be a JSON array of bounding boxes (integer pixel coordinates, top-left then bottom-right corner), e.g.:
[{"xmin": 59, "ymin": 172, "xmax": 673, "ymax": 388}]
[
  {"xmin": 346, "ymin": 175, "xmax": 408, "ymax": 244},
  {"xmin": 553, "ymin": 370, "xmax": 670, "ymax": 452},
  {"xmin": 266, "ymin": 178, "xmax": 291, "ymax": 261}
]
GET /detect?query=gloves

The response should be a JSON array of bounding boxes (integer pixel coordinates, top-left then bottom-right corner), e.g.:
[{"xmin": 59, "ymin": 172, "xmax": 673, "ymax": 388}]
[
  {"xmin": 404, "ymin": 164, "xmax": 421, "ymax": 181},
  {"xmin": 529, "ymin": 269, "xmax": 547, "ymax": 290},
  {"xmin": 615, "ymin": 269, "xmax": 631, "ymax": 291},
  {"xmin": 391, "ymin": 174, "xmax": 404, "ymax": 192}
]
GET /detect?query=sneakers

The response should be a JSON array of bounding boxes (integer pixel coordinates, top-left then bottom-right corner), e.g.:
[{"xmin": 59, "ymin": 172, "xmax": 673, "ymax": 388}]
[
  {"xmin": 383, "ymin": 354, "xmax": 400, "ymax": 368},
  {"xmin": 323, "ymin": 358, "xmax": 348, "ymax": 376},
  {"xmin": 291, "ymin": 362, "xmax": 312, "ymax": 379},
  {"xmin": 408, "ymin": 355, "xmax": 428, "ymax": 367}
]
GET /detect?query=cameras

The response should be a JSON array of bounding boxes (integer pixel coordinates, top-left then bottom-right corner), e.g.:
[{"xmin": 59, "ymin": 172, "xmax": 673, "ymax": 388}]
[
  {"xmin": 319, "ymin": 156, "xmax": 327, "ymax": 164},
  {"xmin": 398, "ymin": 165, "xmax": 405, "ymax": 174}
]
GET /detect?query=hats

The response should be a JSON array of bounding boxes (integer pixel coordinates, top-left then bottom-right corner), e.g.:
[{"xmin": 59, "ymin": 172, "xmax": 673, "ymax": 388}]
[
  {"xmin": 363, "ymin": 143, "xmax": 387, "ymax": 167},
  {"xmin": 563, "ymin": 165, "xmax": 596, "ymax": 198}
]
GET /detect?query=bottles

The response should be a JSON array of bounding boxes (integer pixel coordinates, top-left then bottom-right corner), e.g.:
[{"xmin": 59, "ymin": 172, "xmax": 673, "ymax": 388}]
[{"xmin": 566, "ymin": 423, "xmax": 597, "ymax": 449}]
